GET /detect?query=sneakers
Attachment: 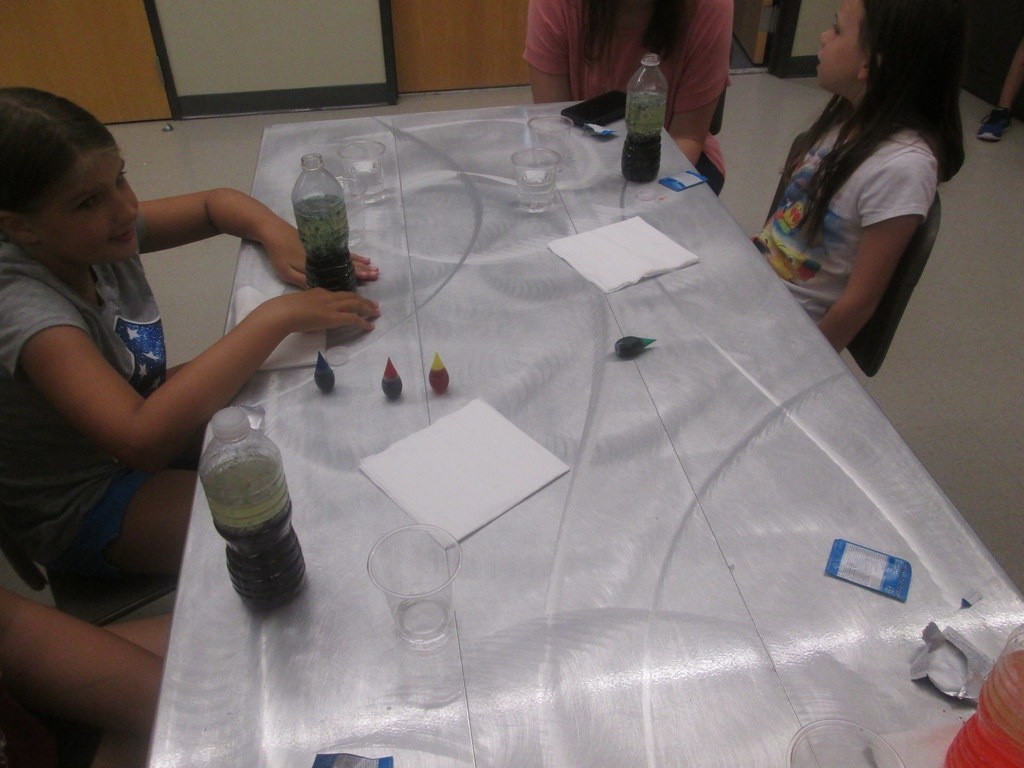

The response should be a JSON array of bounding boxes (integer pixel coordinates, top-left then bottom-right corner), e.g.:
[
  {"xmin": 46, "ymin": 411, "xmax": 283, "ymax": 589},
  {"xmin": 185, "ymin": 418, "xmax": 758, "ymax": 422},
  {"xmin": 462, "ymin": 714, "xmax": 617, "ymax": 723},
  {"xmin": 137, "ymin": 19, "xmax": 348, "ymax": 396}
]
[{"xmin": 977, "ymin": 108, "xmax": 1012, "ymax": 141}]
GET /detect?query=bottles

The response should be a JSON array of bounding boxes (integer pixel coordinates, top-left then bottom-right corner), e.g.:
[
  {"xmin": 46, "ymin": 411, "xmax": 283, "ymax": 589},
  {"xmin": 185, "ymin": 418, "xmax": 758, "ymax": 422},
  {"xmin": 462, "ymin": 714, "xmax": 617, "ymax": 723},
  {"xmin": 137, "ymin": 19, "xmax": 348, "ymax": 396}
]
[
  {"xmin": 198, "ymin": 407, "xmax": 307, "ymax": 612},
  {"xmin": 621, "ymin": 53, "xmax": 669, "ymax": 184},
  {"xmin": 944, "ymin": 623, "xmax": 1024, "ymax": 768},
  {"xmin": 291, "ymin": 153, "xmax": 357, "ymax": 295}
]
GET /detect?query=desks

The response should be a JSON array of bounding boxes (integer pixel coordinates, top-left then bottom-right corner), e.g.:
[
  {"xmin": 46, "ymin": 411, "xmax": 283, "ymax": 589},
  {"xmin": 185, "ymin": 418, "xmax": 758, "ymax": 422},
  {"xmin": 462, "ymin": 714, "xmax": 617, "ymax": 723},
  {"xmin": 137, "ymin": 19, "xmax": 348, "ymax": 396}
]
[{"xmin": 150, "ymin": 102, "xmax": 1024, "ymax": 768}]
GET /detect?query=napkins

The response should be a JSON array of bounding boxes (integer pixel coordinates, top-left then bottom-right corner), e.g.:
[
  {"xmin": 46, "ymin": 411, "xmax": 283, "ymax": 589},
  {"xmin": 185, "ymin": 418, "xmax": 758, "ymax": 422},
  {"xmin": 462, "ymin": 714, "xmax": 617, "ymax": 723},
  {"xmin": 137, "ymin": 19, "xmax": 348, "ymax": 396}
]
[
  {"xmin": 547, "ymin": 217, "xmax": 699, "ymax": 294},
  {"xmin": 356, "ymin": 395, "xmax": 570, "ymax": 550}
]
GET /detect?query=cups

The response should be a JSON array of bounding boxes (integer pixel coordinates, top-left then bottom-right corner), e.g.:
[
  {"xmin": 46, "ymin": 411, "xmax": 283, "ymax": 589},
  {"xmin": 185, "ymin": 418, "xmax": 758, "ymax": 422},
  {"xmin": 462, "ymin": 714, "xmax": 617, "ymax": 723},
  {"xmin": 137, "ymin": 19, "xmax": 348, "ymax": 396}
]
[
  {"xmin": 333, "ymin": 174, "xmax": 368, "ymax": 247},
  {"xmin": 366, "ymin": 522, "xmax": 463, "ymax": 645},
  {"xmin": 510, "ymin": 146, "xmax": 562, "ymax": 214},
  {"xmin": 787, "ymin": 719, "xmax": 906, "ymax": 768},
  {"xmin": 337, "ymin": 139, "xmax": 387, "ymax": 204},
  {"xmin": 526, "ymin": 114, "xmax": 574, "ymax": 172}
]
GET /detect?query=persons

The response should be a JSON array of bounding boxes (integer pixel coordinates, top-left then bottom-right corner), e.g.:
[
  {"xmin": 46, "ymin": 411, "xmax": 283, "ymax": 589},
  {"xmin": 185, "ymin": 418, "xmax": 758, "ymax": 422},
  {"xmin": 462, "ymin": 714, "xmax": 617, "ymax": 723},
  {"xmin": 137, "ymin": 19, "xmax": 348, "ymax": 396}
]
[
  {"xmin": 521, "ymin": 0, "xmax": 735, "ymax": 196},
  {"xmin": 0, "ymin": 586, "xmax": 174, "ymax": 768},
  {"xmin": 976, "ymin": 37, "xmax": 1024, "ymax": 140},
  {"xmin": 750, "ymin": 0, "xmax": 965, "ymax": 353},
  {"xmin": 0, "ymin": 85, "xmax": 383, "ymax": 591}
]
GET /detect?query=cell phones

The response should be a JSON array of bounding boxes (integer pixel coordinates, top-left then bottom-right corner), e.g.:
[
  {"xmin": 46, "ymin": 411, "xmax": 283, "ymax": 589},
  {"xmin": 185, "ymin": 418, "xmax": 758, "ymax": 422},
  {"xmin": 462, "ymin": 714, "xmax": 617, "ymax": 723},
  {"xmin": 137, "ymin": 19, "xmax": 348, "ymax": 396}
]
[{"xmin": 561, "ymin": 90, "xmax": 627, "ymax": 128}]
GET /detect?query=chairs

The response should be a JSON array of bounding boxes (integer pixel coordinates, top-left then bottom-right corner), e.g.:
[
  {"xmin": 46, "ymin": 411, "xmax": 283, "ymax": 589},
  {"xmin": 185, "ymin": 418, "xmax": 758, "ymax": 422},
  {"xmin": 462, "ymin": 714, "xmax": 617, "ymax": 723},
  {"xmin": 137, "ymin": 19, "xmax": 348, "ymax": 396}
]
[
  {"xmin": 0, "ymin": 513, "xmax": 178, "ymax": 627},
  {"xmin": 762, "ymin": 132, "xmax": 942, "ymax": 380}
]
